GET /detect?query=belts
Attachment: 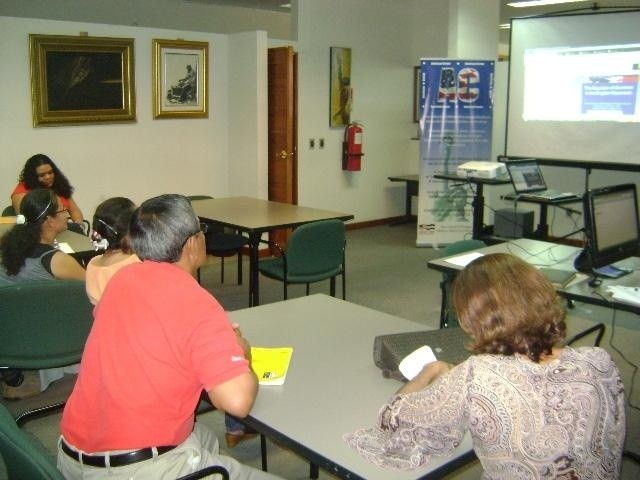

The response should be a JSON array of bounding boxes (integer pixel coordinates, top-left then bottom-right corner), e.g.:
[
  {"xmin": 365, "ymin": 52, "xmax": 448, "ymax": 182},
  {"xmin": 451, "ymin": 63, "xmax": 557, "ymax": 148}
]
[{"xmin": 59, "ymin": 440, "xmax": 177, "ymax": 467}]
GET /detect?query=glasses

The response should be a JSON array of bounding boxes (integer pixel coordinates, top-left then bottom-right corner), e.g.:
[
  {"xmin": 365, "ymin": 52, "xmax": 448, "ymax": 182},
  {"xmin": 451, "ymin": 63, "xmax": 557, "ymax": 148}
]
[{"xmin": 183, "ymin": 223, "xmax": 209, "ymax": 247}]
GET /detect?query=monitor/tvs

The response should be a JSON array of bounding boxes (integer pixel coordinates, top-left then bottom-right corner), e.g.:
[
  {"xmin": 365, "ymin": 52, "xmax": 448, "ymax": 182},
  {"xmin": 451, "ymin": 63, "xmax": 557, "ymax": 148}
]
[{"xmin": 574, "ymin": 183, "xmax": 640, "ymax": 279}]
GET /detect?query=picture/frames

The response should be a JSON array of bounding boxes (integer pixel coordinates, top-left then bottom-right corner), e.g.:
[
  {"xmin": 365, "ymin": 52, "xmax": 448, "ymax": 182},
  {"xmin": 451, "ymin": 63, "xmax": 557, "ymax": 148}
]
[
  {"xmin": 414, "ymin": 66, "xmax": 422, "ymax": 124},
  {"xmin": 28, "ymin": 34, "xmax": 138, "ymax": 128},
  {"xmin": 152, "ymin": 39, "xmax": 209, "ymax": 119}
]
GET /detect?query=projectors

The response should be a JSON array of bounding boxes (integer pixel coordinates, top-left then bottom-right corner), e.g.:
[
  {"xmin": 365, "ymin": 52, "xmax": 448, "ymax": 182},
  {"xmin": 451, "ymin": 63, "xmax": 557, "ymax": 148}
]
[{"xmin": 456, "ymin": 159, "xmax": 507, "ymax": 182}]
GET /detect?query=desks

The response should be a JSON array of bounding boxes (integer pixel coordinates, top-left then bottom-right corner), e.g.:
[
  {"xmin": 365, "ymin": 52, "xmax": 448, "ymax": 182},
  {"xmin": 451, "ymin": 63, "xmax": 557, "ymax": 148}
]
[
  {"xmin": 0, "ymin": 223, "xmax": 106, "ymax": 272},
  {"xmin": 434, "ymin": 175, "xmax": 512, "ymax": 246},
  {"xmin": 191, "ymin": 197, "xmax": 354, "ymax": 307},
  {"xmin": 200, "ymin": 293, "xmax": 476, "ymax": 480},
  {"xmin": 387, "ymin": 175, "xmax": 419, "ymax": 227},
  {"xmin": 427, "ymin": 238, "xmax": 640, "ymax": 314},
  {"xmin": 500, "ymin": 194, "xmax": 584, "ymax": 241}
]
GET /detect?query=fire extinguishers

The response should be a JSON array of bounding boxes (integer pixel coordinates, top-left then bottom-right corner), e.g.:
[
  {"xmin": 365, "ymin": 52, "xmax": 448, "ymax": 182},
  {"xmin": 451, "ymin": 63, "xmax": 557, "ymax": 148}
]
[{"xmin": 342, "ymin": 121, "xmax": 364, "ymax": 171}]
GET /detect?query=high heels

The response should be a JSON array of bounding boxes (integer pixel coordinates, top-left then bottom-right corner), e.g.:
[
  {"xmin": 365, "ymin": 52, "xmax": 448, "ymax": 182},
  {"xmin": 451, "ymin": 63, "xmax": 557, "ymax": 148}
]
[{"xmin": 225, "ymin": 427, "xmax": 257, "ymax": 447}]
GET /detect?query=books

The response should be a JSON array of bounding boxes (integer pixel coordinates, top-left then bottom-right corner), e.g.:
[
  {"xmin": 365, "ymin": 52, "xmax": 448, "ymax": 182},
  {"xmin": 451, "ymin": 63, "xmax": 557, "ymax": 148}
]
[
  {"xmin": 539, "ymin": 265, "xmax": 577, "ymax": 290},
  {"xmin": 251, "ymin": 343, "xmax": 295, "ymax": 387}
]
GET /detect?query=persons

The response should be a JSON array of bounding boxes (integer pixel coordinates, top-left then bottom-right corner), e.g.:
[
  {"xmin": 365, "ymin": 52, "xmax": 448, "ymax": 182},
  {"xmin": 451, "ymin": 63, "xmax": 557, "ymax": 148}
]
[
  {"xmin": 333, "ymin": 54, "xmax": 351, "ymax": 125},
  {"xmin": 84, "ymin": 197, "xmax": 143, "ymax": 307},
  {"xmin": 0, "ymin": 189, "xmax": 87, "ymax": 402},
  {"xmin": 11, "ymin": 153, "xmax": 85, "ymax": 233},
  {"xmin": 430, "ymin": 142, "xmax": 470, "ymax": 224},
  {"xmin": 177, "ymin": 63, "xmax": 196, "ymax": 102},
  {"xmin": 55, "ymin": 193, "xmax": 287, "ymax": 480},
  {"xmin": 345, "ymin": 252, "xmax": 628, "ymax": 479}
]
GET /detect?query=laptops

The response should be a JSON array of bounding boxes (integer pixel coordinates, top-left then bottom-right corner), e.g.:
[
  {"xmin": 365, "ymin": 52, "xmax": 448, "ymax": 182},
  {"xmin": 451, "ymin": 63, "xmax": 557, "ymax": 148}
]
[{"xmin": 504, "ymin": 160, "xmax": 577, "ymax": 202}]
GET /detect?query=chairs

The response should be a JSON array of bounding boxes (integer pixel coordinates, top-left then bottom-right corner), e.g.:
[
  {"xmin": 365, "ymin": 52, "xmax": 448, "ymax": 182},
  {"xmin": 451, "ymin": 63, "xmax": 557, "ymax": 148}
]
[
  {"xmin": 249, "ymin": 219, "xmax": 346, "ymax": 307},
  {"xmin": 188, "ymin": 196, "xmax": 249, "ymax": 285},
  {"xmin": 0, "ymin": 401, "xmax": 229, "ymax": 480},
  {"xmin": 0, "ymin": 280, "xmax": 96, "ymax": 370},
  {"xmin": 440, "ymin": 240, "xmax": 489, "ymax": 329},
  {"xmin": 2, "ymin": 206, "xmax": 90, "ymax": 237}
]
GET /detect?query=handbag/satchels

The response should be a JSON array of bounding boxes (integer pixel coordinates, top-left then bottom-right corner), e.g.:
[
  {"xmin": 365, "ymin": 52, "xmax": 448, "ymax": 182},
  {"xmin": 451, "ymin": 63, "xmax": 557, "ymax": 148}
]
[{"xmin": 373, "ymin": 326, "xmax": 471, "ymax": 383}]
